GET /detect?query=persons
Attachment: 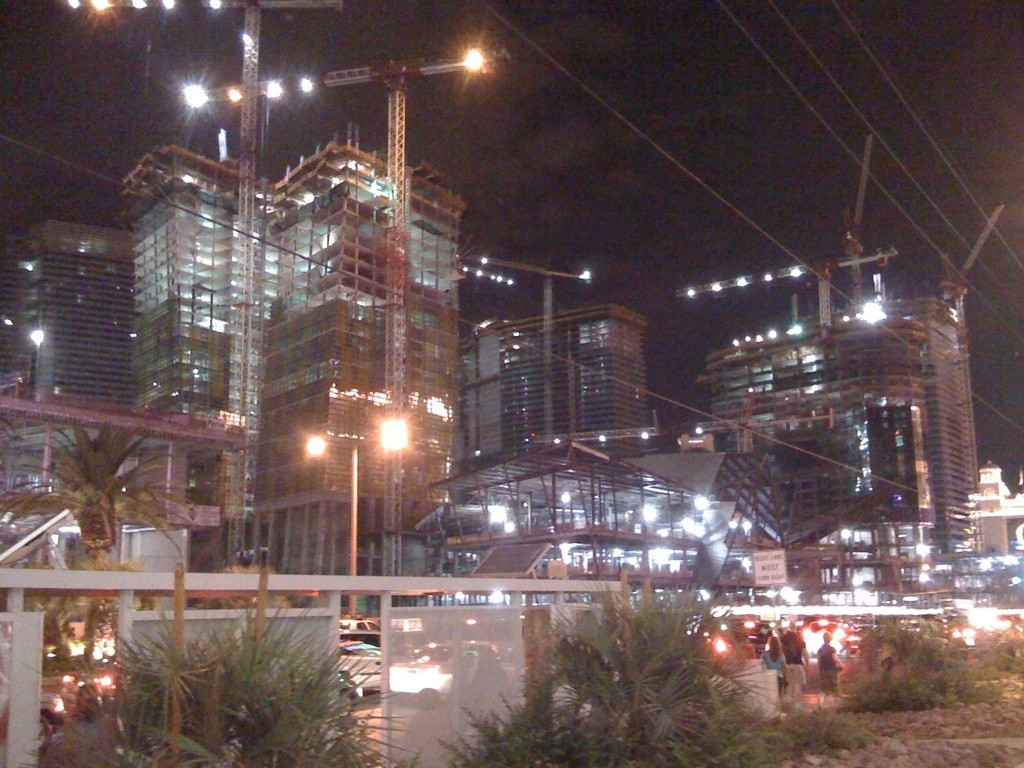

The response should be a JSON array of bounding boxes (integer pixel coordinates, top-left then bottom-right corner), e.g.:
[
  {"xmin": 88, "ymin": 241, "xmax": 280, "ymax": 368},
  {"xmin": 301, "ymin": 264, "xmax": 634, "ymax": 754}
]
[
  {"xmin": 397, "ymin": 645, "xmax": 512, "ymax": 768},
  {"xmin": 37, "ymin": 682, "xmax": 163, "ymax": 768},
  {"xmin": 736, "ymin": 620, "xmax": 876, "ymax": 707}
]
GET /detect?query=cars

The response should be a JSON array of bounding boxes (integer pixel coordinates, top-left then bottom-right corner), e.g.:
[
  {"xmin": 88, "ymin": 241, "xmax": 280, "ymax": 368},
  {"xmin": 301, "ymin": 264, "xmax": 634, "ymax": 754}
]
[
  {"xmin": 702, "ymin": 615, "xmax": 977, "ymax": 669},
  {"xmin": 338, "ymin": 617, "xmax": 504, "ymax": 699},
  {"xmin": 0, "ymin": 647, "xmax": 120, "ymax": 757}
]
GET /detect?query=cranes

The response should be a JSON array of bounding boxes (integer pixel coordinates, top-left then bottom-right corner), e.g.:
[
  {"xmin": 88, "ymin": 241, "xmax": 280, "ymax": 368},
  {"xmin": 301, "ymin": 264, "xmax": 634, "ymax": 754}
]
[
  {"xmin": 676, "ymin": 131, "xmax": 898, "ymax": 306},
  {"xmin": 939, "ymin": 202, "xmax": 1006, "ymax": 305},
  {"xmin": 177, "ymin": 51, "xmax": 490, "ymax": 575},
  {"xmin": 69, "ymin": 0, "xmax": 343, "ymax": 564}
]
